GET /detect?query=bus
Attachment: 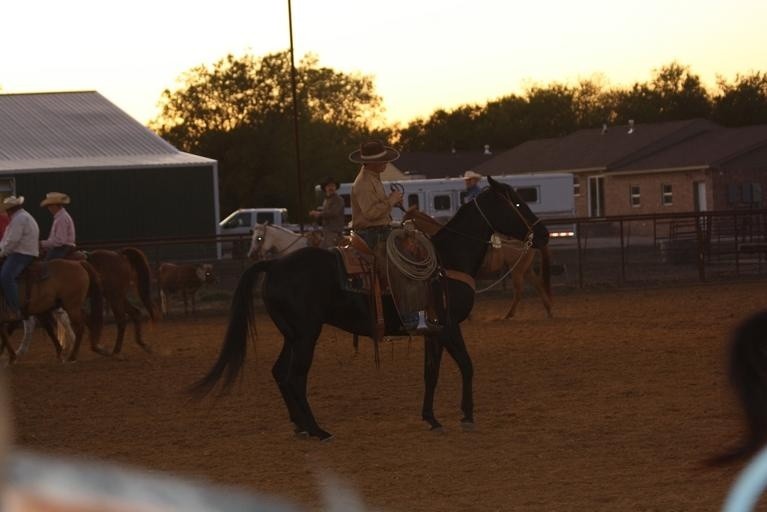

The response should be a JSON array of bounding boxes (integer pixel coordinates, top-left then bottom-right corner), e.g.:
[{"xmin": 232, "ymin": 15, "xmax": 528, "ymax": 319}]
[{"xmin": 315, "ymin": 173, "xmax": 577, "ymax": 238}]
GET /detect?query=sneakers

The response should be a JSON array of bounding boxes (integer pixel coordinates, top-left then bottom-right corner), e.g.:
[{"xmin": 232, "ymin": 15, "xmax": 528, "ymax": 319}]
[
  {"xmin": 8, "ymin": 309, "xmax": 21, "ymax": 321},
  {"xmin": 400, "ymin": 317, "xmax": 444, "ymax": 332}
]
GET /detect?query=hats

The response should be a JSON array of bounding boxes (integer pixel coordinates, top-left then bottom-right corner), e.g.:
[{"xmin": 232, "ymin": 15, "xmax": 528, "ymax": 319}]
[
  {"xmin": 349, "ymin": 140, "xmax": 400, "ymax": 164},
  {"xmin": 321, "ymin": 177, "xmax": 339, "ymax": 192},
  {"xmin": 464, "ymin": 171, "xmax": 481, "ymax": 180},
  {"xmin": 0, "ymin": 195, "xmax": 25, "ymax": 210},
  {"xmin": 40, "ymin": 191, "xmax": 71, "ymax": 207}
]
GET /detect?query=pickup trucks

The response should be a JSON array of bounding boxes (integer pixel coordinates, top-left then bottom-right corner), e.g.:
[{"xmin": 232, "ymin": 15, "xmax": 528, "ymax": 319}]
[{"xmin": 219, "ymin": 208, "xmax": 328, "ymax": 260}]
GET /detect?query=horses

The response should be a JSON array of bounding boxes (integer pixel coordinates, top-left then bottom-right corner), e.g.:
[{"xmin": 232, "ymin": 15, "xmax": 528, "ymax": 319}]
[
  {"xmin": 247, "ymin": 220, "xmax": 352, "ymax": 262},
  {"xmin": 185, "ymin": 174, "xmax": 549, "ymax": 444},
  {"xmin": 15, "ymin": 306, "xmax": 76, "ymax": 360},
  {"xmin": 36, "ymin": 247, "xmax": 158, "ymax": 361},
  {"xmin": 402, "ymin": 205, "xmax": 554, "ymax": 322},
  {"xmin": 0, "ymin": 257, "xmax": 103, "ymax": 364}
]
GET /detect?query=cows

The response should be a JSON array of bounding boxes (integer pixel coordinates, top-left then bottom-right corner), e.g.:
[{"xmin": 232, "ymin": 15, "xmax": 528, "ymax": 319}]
[{"xmin": 159, "ymin": 262, "xmax": 217, "ymax": 318}]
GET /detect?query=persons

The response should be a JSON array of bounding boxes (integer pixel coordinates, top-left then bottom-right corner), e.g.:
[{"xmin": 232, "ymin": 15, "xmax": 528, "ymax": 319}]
[
  {"xmin": 0, "ymin": 195, "xmax": 39, "ymax": 321},
  {"xmin": 40, "ymin": 192, "xmax": 76, "ymax": 260},
  {"xmin": 460, "ymin": 170, "xmax": 482, "ymax": 205},
  {"xmin": 349, "ymin": 140, "xmax": 445, "ymax": 336},
  {"xmin": 308, "ymin": 177, "xmax": 345, "ymax": 249}
]
